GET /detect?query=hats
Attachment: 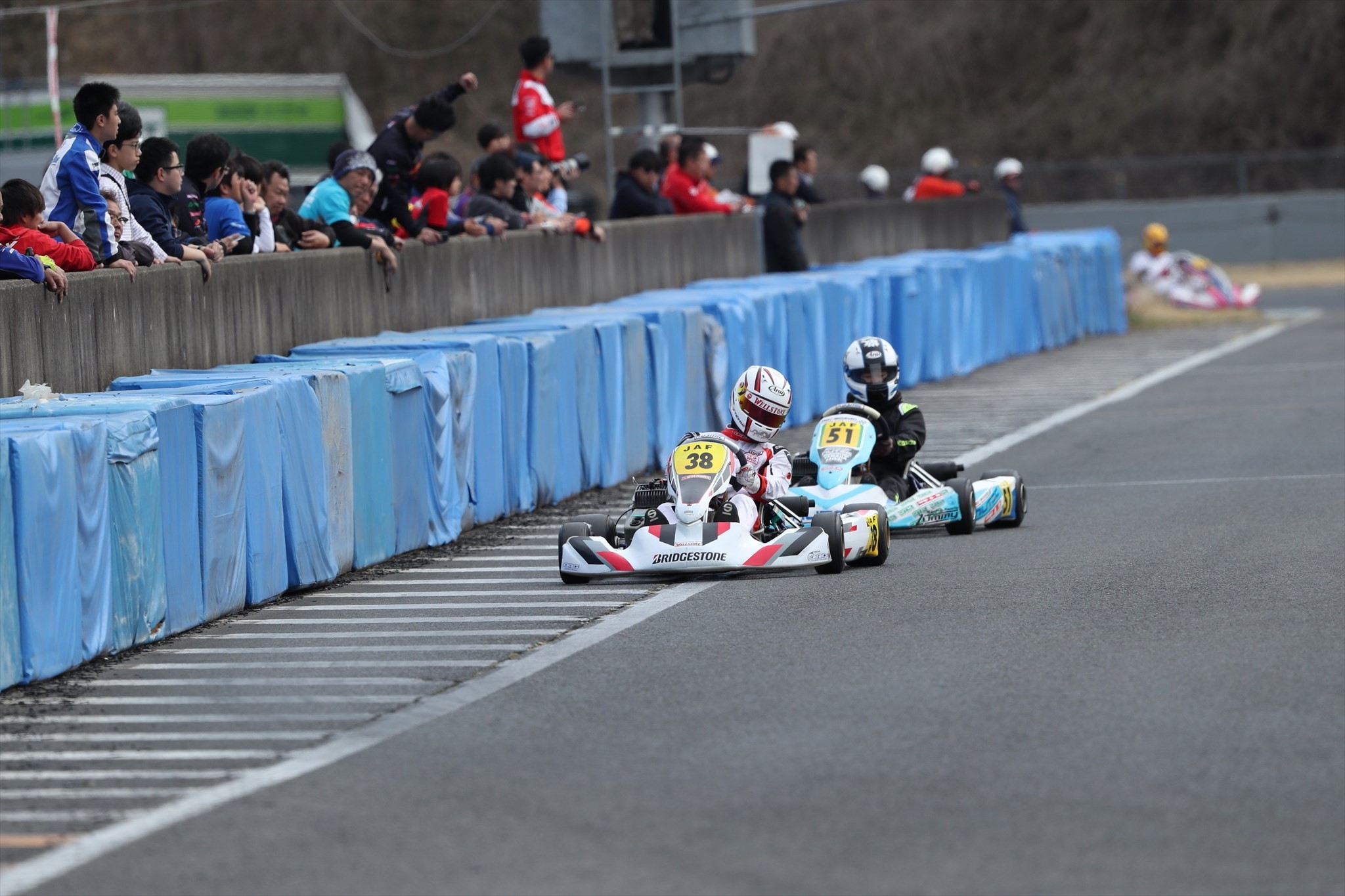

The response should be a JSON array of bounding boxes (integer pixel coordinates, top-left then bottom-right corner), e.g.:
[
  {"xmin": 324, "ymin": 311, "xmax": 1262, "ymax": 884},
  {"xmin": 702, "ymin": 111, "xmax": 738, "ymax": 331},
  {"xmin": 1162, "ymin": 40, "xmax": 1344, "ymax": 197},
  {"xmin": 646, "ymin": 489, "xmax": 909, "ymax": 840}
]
[{"xmin": 334, "ymin": 149, "xmax": 377, "ymax": 186}]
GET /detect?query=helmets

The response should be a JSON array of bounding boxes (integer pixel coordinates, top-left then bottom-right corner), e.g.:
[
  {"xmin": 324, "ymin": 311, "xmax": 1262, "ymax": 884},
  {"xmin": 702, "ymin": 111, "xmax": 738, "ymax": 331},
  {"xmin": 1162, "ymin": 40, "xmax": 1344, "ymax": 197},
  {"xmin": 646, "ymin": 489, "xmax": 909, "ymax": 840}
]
[
  {"xmin": 1142, "ymin": 222, "xmax": 1168, "ymax": 249},
  {"xmin": 842, "ymin": 336, "xmax": 901, "ymax": 406},
  {"xmin": 730, "ymin": 366, "xmax": 792, "ymax": 443},
  {"xmin": 921, "ymin": 147, "xmax": 959, "ymax": 176},
  {"xmin": 860, "ymin": 164, "xmax": 892, "ymax": 193},
  {"xmin": 993, "ymin": 157, "xmax": 1025, "ymax": 181}
]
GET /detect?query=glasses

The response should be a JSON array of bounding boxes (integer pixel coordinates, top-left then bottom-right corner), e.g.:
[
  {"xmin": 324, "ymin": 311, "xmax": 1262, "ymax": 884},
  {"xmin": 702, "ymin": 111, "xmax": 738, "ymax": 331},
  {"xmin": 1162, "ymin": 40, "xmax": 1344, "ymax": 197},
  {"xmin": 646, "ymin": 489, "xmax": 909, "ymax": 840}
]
[
  {"xmin": 164, "ymin": 164, "xmax": 184, "ymax": 173},
  {"xmin": 222, "ymin": 167, "xmax": 230, "ymax": 175},
  {"xmin": 122, "ymin": 142, "xmax": 141, "ymax": 151},
  {"xmin": 109, "ymin": 214, "xmax": 128, "ymax": 224}
]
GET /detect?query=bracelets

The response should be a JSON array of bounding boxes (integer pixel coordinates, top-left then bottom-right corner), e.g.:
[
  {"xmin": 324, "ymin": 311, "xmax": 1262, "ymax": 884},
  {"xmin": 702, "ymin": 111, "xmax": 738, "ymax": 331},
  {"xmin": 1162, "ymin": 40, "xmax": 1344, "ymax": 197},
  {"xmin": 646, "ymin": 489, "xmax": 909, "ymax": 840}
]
[
  {"xmin": 44, "ymin": 265, "xmax": 51, "ymax": 271},
  {"xmin": 197, "ymin": 245, "xmax": 202, "ymax": 249}
]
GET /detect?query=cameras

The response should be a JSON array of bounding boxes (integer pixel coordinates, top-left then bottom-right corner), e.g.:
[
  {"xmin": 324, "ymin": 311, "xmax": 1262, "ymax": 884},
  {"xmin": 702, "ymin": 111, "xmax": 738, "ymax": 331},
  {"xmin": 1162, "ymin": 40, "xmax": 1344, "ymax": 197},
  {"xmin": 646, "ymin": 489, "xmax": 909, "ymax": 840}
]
[{"xmin": 550, "ymin": 151, "xmax": 592, "ymax": 174}]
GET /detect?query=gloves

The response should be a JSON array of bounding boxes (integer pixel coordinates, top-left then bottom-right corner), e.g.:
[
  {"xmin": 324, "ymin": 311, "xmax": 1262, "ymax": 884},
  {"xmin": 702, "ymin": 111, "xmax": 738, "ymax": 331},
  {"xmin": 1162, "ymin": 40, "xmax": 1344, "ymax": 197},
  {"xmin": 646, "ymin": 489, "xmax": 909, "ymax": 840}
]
[
  {"xmin": 875, "ymin": 433, "xmax": 895, "ymax": 459},
  {"xmin": 736, "ymin": 464, "xmax": 756, "ymax": 488}
]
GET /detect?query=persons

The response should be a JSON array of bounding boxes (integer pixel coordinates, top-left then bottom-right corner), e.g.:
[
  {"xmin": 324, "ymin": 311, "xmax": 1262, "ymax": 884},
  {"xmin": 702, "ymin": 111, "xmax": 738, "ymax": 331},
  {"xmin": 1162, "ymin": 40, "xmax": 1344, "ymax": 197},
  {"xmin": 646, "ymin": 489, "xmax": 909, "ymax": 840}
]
[
  {"xmin": 902, "ymin": 146, "xmax": 983, "ymax": 203},
  {"xmin": 0, "ymin": 73, "xmax": 607, "ymax": 304},
  {"xmin": 797, "ymin": 336, "xmax": 926, "ymax": 502},
  {"xmin": 645, "ymin": 365, "xmax": 793, "ymax": 532},
  {"xmin": 993, "ymin": 157, "xmax": 1029, "ymax": 233},
  {"xmin": 859, "ymin": 165, "xmax": 890, "ymax": 198},
  {"xmin": 511, "ymin": 36, "xmax": 574, "ymax": 163},
  {"xmin": 764, "ymin": 160, "xmax": 810, "ymax": 273},
  {"xmin": 1130, "ymin": 223, "xmax": 1175, "ymax": 280},
  {"xmin": 609, "ymin": 121, "xmax": 830, "ymax": 221}
]
